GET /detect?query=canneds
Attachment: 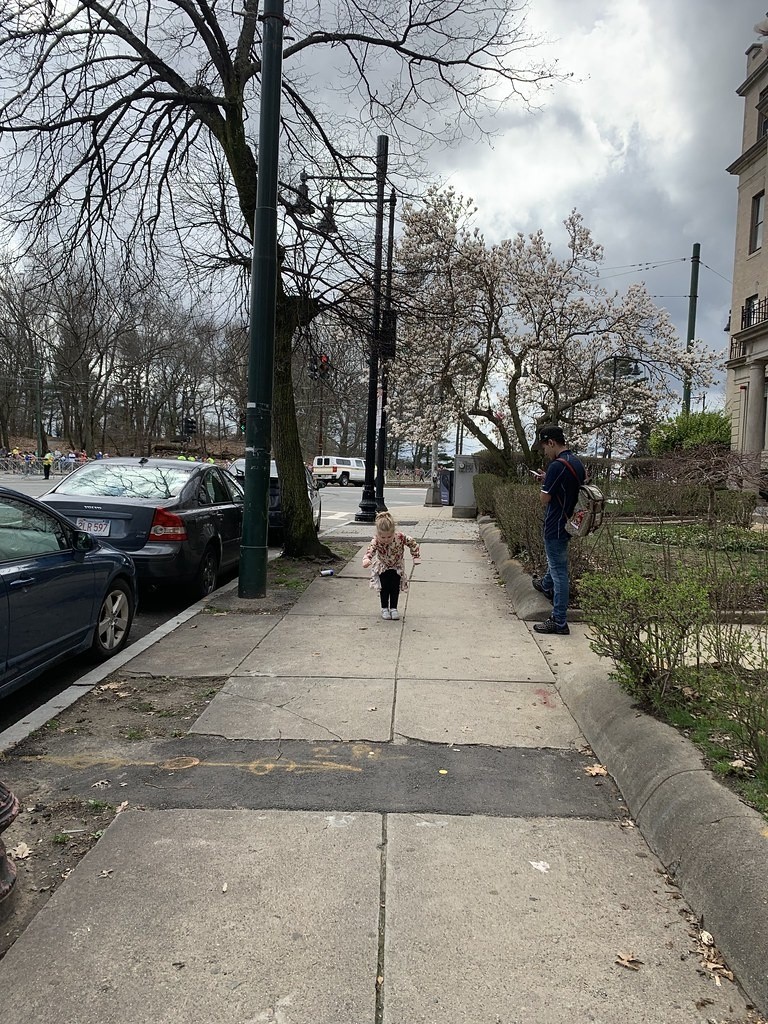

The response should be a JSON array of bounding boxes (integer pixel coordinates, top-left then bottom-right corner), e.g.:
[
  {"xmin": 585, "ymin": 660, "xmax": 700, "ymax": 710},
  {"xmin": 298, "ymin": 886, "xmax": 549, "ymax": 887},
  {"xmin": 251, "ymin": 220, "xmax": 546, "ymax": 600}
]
[{"xmin": 319, "ymin": 569, "xmax": 334, "ymax": 576}]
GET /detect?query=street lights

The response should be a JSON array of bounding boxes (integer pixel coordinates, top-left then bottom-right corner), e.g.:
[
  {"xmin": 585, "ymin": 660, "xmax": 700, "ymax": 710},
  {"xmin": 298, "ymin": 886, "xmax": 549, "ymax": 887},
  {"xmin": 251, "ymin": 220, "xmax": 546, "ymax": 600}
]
[
  {"xmin": 21, "ymin": 357, "xmax": 42, "ymax": 460},
  {"xmin": 602, "ymin": 354, "xmax": 643, "ymax": 497},
  {"xmin": 289, "ymin": 134, "xmax": 388, "ymax": 525},
  {"xmin": 318, "ymin": 189, "xmax": 396, "ymax": 513}
]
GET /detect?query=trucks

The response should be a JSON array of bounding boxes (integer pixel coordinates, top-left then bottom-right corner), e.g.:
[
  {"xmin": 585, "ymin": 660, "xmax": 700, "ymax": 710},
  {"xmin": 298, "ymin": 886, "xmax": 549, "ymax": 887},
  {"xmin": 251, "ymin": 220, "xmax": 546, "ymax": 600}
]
[{"xmin": 312, "ymin": 455, "xmax": 387, "ymax": 486}]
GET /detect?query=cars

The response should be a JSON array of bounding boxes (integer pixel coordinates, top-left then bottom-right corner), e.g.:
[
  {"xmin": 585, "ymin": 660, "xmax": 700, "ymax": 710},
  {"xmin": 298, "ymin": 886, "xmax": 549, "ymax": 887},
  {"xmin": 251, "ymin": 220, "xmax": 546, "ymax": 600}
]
[
  {"xmin": 0, "ymin": 486, "xmax": 139, "ymax": 700},
  {"xmin": 34, "ymin": 456, "xmax": 246, "ymax": 606},
  {"xmin": 227, "ymin": 458, "xmax": 321, "ymax": 543},
  {"xmin": 170, "ymin": 435, "xmax": 192, "ymax": 443}
]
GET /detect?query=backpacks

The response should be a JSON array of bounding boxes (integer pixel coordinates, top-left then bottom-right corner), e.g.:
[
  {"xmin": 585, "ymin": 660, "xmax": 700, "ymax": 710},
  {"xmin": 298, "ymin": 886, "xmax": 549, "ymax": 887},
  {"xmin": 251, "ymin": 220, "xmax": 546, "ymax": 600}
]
[{"xmin": 555, "ymin": 455, "xmax": 605, "ymax": 537}]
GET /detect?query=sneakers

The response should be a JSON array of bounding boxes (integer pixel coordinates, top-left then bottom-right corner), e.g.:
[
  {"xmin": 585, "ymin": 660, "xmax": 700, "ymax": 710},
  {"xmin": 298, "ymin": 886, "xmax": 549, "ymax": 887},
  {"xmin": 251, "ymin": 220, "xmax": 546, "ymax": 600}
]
[
  {"xmin": 534, "ymin": 612, "xmax": 570, "ymax": 635},
  {"xmin": 532, "ymin": 578, "xmax": 553, "ymax": 599},
  {"xmin": 381, "ymin": 607, "xmax": 391, "ymax": 620},
  {"xmin": 390, "ymin": 608, "xmax": 399, "ymax": 619}
]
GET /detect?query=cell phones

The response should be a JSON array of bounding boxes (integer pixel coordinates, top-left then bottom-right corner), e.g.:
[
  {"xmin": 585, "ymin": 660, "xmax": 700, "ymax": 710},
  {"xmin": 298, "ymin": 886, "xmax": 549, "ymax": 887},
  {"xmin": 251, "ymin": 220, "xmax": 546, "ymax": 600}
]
[{"xmin": 528, "ymin": 470, "xmax": 542, "ymax": 478}]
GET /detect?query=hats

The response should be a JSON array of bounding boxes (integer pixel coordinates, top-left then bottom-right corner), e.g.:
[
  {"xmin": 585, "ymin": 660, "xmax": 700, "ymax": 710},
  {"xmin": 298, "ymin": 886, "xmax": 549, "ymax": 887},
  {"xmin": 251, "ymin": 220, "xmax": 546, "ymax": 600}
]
[{"xmin": 538, "ymin": 426, "xmax": 563, "ymax": 442}]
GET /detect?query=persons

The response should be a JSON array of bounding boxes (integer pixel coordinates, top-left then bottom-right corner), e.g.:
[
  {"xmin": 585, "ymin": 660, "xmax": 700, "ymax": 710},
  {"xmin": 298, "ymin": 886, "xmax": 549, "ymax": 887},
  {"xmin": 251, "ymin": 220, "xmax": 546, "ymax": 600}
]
[
  {"xmin": 81, "ymin": 450, "xmax": 87, "ymax": 459},
  {"xmin": 13, "ymin": 446, "xmax": 36, "ymax": 474},
  {"xmin": 95, "ymin": 451, "xmax": 108, "ymax": 460},
  {"xmin": 41, "ymin": 449, "xmax": 52, "ymax": 479},
  {"xmin": 419, "ymin": 468, "xmax": 425, "ymax": 482},
  {"xmin": 178, "ymin": 453, "xmax": 214, "ymax": 464},
  {"xmin": 226, "ymin": 458, "xmax": 236, "ymax": 467},
  {"xmin": 362, "ymin": 511, "xmax": 421, "ymax": 621},
  {"xmin": 532, "ymin": 426, "xmax": 585, "ymax": 634}
]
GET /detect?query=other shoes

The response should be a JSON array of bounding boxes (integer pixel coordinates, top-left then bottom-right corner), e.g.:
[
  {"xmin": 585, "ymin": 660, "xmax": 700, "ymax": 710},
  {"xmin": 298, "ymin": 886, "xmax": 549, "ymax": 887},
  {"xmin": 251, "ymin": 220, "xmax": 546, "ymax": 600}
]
[{"xmin": 44, "ymin": 477, "xmax": 49, "ymax": 479}]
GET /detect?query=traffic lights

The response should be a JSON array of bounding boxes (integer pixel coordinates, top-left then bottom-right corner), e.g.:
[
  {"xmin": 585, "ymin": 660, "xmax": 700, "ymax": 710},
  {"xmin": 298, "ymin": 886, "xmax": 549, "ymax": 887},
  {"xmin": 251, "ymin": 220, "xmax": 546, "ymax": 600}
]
[
  {"xmin": 191, "ymin": 417, "xmax": 197, "ymax": 433},
  {"xmin": 320, "ymin": 354, "xmax": 330, "ymax": 379},
  {"xmin": 239, "ymin": 413, "xmax": 246, "ymax": 431},
  {"xmin": 307, "ymin": 355, "xmax": 318, "ymax": 381},
  {"xmin": 186, "ymin": 417, "xmax": 192, "ymax": 433}
]
